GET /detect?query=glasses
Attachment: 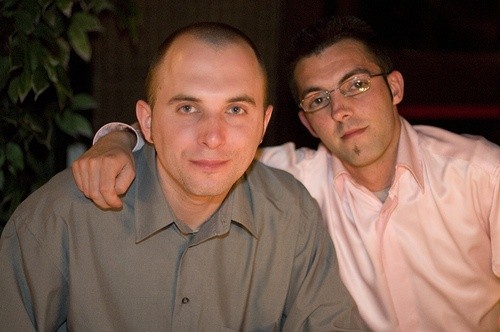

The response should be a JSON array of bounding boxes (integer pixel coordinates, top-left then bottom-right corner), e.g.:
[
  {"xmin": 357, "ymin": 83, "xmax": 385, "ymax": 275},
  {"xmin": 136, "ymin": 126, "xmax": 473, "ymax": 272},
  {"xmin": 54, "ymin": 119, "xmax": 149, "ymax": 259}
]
[{"xmin": 298, "ymin": 73, "xmax": 385, "ymax": 113}]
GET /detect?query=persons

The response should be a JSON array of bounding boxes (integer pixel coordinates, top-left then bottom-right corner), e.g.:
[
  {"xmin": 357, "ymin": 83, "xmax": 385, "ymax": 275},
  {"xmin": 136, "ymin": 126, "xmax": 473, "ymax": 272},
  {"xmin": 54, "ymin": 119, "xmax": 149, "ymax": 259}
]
[
  {"xmin": 0, "ymin": 23, "xmax": 374, "ymax": 332},
  {"xmin": 74, "ymin": 23, "xmax": 500, "ymax": 332}
]
